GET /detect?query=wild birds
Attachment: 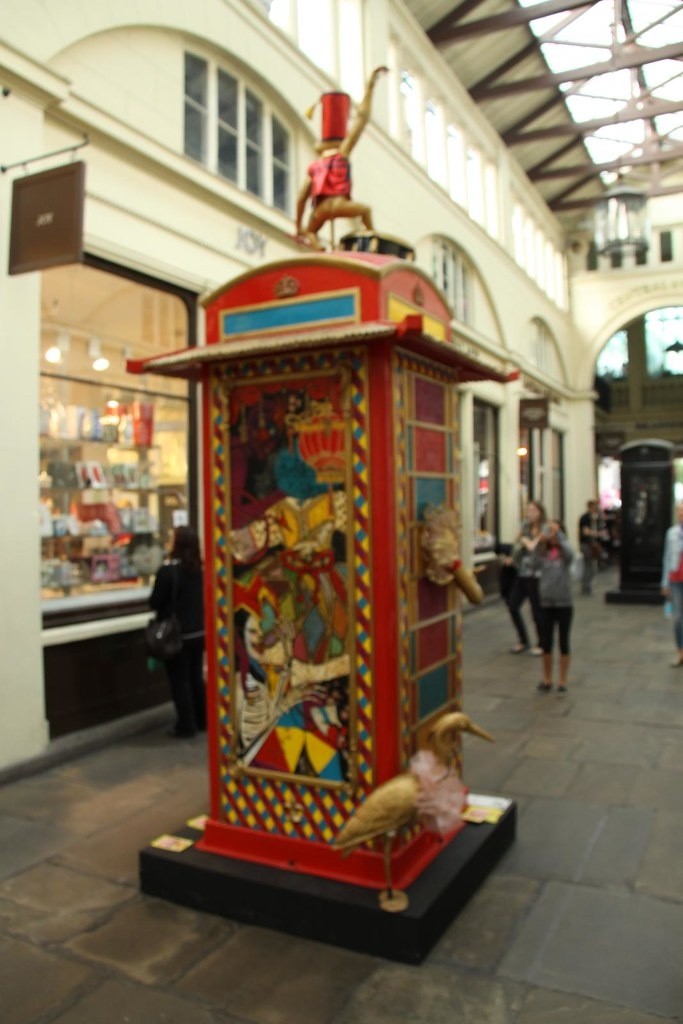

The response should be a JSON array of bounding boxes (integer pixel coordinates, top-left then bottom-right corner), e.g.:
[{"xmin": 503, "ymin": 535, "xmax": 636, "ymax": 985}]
[{"xmin": 332, "ymin": 712, "xmax": 496, "ymax": 913}]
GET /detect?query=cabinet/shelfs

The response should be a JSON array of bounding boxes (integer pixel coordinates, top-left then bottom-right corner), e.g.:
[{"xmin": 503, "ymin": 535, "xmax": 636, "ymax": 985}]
[{"xmin": 39, "ymin": 434, "xmax": 162, "ymax": 600}]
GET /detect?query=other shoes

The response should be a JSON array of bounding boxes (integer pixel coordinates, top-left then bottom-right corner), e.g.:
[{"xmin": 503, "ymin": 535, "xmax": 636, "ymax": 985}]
[
  {"xmin": 531, "ymin": 647, "xmax": 544, "ymax": 656},
  {"xmin": 534, "ymin": 682, "xmax": 551, "ymax": 695},
  {"xmin": 510, "ymin": 644, "xmax": 529, "ymax": 653},
  {"xmin": 169, "ymin": 724, "xmax": 180, "ymax": 736},
  {"xmin": 671, "ymin": 658, "xmax": 683, "ymax": 667},
  {"xmin": 556, "ymin": 685, "xmax": 567, "ymax": 698}
]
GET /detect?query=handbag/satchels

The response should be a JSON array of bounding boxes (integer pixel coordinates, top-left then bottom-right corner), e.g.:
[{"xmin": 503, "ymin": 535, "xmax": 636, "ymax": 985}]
[{"xmin": 146, "ymin": 619, "xmax": 183, "ymax": 656}]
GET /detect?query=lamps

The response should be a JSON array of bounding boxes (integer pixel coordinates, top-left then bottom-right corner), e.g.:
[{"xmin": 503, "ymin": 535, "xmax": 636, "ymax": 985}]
[
  {"xmin": 87, "ymin": 339, "xmax": 110, "ymax": 372},
  {"xmin": 44, "ymin": 331, "xmax": 72, "ymax": 364}
]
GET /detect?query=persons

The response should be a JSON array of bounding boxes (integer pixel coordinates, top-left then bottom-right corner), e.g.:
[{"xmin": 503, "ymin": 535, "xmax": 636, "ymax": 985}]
[
  {"xmin": 500, "ymin": 503, "xmax": 544, "ymax": 656},
  {"xmin": 147, "ymin": 525, "xmax": 206, "ymax": 739},
  {"xmin": 294, "ymin": 65, "xmax": 389, "ymax": 252},
  {"xmin": 662, "ymin": 499, "xmax": 683, "ymax": 666},
  {"xmin": 613, "ymin": 489, "xmax": 622, "ymax": 567},
  {"xmin": 580, "ymin": 500, "xmax": 608, "ymax": 596},
  {"xmin": 533, "ymin": 522, "xmax": 576, "ymax": 694}
]
